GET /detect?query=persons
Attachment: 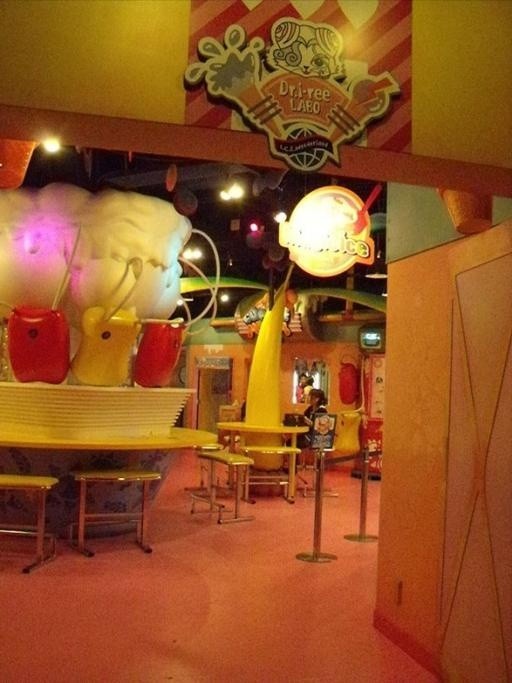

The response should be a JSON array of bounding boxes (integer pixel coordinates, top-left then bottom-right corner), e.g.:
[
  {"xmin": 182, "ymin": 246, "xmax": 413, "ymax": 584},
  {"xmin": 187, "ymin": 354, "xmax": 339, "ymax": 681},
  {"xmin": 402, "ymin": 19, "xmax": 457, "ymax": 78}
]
[
  {"xmin": 281, "ymin": 388, "xmax": 330, "ymax": 474},
  {"xmin": 295, "ymin": 372, "xmax": 306, "ymax": 403},
  {"xmin": 302, "ymin": 375, "xmax": 314, "ymax": 403}
]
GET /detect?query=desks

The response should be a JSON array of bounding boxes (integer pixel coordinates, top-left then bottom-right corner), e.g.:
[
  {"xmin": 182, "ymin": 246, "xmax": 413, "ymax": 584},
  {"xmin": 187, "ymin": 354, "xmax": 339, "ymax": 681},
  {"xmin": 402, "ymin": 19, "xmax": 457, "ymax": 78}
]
[
  {"xmin": 216, "ymin": 419, "xmax": 310, "ymax": 504},
  {"xmin": 1, "ymin": 426, "xmax": 218, "ymax": 455}
]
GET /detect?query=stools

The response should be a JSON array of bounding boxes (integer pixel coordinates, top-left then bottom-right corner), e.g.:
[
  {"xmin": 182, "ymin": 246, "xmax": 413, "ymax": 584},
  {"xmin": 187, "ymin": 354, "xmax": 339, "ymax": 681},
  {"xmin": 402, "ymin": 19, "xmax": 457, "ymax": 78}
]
[
  {"xmin": 189, "ymin": 440, "xmax": 302, "ymax": 525},
  {"xmin": 1, "ymin": 464, "xmax": 163, "ymax": 574}
]
